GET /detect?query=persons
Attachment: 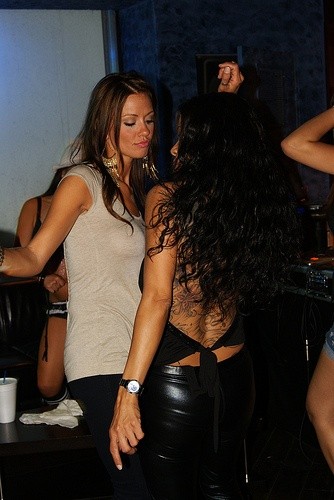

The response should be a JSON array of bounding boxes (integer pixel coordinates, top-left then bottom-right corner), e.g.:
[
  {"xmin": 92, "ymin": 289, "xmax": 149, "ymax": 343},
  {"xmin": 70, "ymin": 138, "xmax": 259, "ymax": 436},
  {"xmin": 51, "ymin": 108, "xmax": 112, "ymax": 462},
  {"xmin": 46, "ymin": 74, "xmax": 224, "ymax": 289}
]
[
  {"xmin": 0, "ymin": 59, "xmax": 245, "ymax": 500},
  {"xmin": 108, "ymin": 92, "xmax": 306, "ymax": 500},
  {"xmin": 281, "ymin": 104, "xmax": 334, "ymax": 500},
  {"xmin": 13, "ymin": 138, "xmax": 93, "ymax": 409}
]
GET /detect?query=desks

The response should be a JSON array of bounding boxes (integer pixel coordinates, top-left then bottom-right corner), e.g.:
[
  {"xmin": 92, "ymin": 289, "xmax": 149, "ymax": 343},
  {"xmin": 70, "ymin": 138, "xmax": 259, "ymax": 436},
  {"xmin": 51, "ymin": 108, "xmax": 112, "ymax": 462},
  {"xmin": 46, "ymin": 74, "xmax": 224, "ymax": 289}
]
[{"xmin": 0, "ymin": 400, "xmax": 98, "ymax": 456}]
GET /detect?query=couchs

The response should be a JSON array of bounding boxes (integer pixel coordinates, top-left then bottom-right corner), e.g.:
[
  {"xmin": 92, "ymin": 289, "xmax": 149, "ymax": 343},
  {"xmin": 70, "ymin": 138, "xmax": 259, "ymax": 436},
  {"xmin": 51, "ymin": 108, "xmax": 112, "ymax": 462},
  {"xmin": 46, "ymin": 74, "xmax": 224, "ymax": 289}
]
[{"xmin": 0, "ymin": 276, "xmax": 52, "ymax": 394}]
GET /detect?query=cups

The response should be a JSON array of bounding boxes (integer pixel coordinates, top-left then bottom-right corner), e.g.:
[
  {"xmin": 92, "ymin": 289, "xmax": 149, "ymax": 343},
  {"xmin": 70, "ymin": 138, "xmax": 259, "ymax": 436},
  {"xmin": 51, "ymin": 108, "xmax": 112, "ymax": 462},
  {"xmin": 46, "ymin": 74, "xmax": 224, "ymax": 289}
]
[{"xmin": 0, "ymin": 377, "xmax": 18, "ymax": 424}]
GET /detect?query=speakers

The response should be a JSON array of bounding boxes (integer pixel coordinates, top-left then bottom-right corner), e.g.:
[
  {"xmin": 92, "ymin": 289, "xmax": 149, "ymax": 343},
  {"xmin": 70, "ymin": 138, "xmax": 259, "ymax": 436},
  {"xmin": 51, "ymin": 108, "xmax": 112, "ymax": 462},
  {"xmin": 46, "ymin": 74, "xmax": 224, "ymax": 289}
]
[{"xmin": 272, "ymin": 284, "xmax": 334, "ymax": 453}]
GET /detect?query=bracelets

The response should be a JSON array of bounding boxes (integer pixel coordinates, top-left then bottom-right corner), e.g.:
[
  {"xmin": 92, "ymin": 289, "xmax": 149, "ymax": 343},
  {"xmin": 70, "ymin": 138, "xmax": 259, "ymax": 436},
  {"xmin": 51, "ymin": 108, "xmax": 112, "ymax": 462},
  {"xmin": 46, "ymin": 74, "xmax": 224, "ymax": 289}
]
[
  {"xmin": 0, "ymin": 245, "xmax": 5, "ymax": 267},
  {"xmin": 38, "ymin": 272, "xmax": 48, "ymax": 286}
]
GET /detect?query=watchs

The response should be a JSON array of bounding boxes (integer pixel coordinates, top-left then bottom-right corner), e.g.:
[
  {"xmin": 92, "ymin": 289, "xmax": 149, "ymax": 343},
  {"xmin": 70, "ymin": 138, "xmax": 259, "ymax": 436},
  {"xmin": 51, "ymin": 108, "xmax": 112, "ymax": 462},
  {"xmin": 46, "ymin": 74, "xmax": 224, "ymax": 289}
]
[{"xmin": 119, "ymin": 377, "xmax": 142, "ymax": 395}]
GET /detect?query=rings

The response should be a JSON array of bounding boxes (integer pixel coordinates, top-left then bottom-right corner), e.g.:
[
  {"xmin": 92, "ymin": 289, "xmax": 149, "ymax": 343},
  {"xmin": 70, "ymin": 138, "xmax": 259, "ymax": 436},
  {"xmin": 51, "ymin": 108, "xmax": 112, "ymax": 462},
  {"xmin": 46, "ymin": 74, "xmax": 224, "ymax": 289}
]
[{"xmin": 221, "ymin": 81, "xmax": 228, "ymax": 86}]
